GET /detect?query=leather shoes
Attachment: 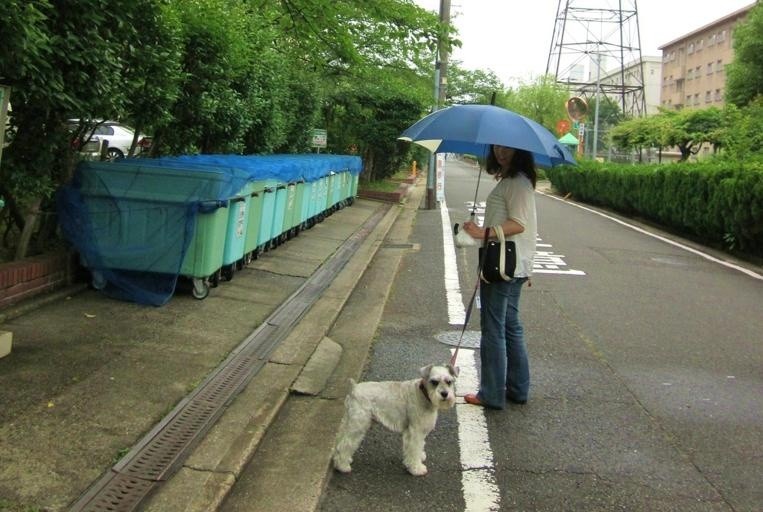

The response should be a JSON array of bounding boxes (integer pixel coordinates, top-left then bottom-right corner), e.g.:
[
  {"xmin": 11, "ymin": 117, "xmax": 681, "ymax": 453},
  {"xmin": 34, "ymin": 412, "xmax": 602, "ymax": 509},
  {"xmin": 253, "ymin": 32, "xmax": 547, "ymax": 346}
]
[{"xmin": 464, "ymin": 394, "xmax": 502, "ymax": 410}]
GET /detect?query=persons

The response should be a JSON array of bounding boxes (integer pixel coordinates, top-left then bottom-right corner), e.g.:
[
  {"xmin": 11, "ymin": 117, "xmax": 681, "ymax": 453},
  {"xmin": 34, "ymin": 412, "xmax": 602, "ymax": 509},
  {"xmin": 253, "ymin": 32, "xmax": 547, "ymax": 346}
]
[{"xmin": 461, "ymin": 142, "xmax": 537, "ymax": 413}]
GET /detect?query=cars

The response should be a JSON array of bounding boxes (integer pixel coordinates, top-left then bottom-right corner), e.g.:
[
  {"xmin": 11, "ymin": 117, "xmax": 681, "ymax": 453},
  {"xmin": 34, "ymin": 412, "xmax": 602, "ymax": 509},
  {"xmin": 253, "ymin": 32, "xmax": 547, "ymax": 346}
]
[{"xmin": 3, "ymin": 118, "xmax": 156, "ymax": 164}]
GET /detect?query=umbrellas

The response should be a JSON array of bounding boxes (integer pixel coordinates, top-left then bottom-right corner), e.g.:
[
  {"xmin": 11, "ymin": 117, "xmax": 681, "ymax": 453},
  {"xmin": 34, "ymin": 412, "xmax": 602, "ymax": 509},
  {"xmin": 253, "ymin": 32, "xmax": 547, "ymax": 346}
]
[{"xmin": 397, "ymin": 91, "xmax": 578, "ymax": 239}]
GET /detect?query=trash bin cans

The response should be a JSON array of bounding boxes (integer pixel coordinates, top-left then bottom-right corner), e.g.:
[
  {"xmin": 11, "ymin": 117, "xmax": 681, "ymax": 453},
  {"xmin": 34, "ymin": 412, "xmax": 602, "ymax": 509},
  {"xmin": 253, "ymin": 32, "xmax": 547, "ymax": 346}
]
[{"xmin": 78, "ymin": 154, "xmax": 362, "ymax": 299}]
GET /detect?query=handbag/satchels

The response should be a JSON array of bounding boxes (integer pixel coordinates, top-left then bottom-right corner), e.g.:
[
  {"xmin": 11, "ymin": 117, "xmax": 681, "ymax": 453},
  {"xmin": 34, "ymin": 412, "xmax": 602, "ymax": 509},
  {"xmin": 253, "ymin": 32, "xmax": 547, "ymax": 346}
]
[{"xmin": 479, "ymin": 241, "xmax": 517, "ymax": 286}]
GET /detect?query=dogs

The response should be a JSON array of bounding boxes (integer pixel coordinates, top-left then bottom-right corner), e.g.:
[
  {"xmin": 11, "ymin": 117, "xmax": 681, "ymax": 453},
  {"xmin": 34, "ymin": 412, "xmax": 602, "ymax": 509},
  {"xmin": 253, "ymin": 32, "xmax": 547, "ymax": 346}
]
[{"xmin": 330, "ymin": 362, "xmax": 461, "ymax": 477}]
